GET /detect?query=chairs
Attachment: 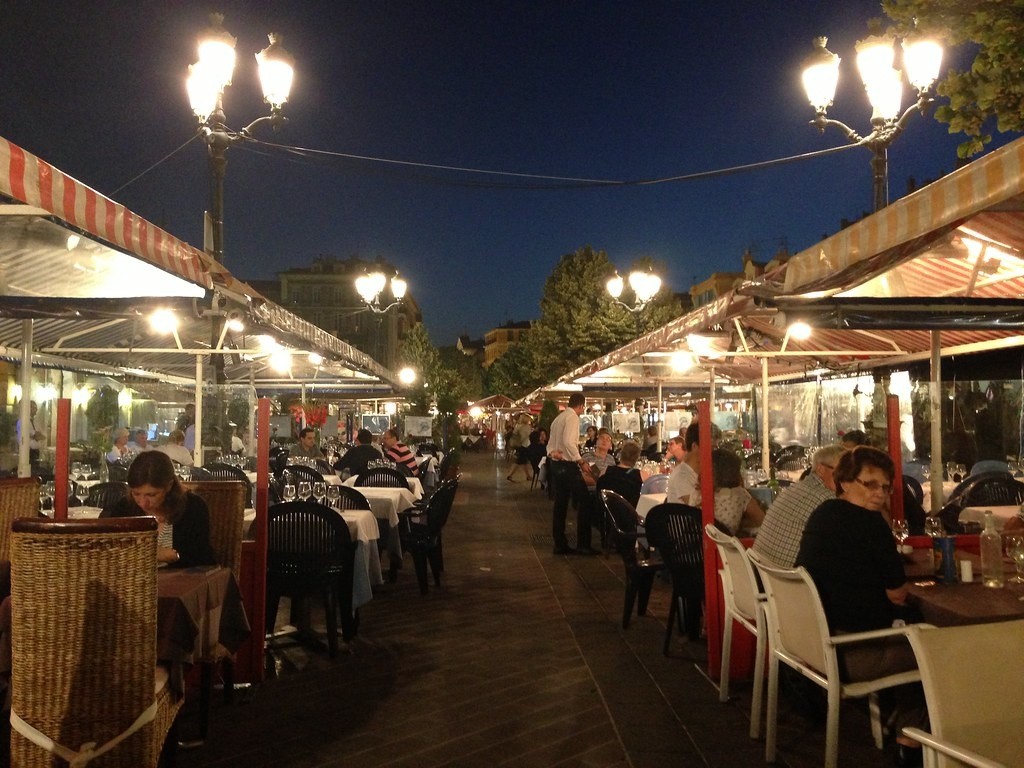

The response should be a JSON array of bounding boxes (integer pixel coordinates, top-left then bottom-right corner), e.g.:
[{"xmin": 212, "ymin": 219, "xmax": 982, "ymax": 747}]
[
  {"xmin": 0, "ymin": 444, "xmax": 461, "ymax": 768},
  {"xmin": 523, "ymin": 440, "xmax": 1024, "ymax": 768}
]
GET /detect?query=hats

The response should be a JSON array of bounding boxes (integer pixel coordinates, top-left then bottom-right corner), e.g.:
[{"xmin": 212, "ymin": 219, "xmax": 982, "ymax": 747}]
[{"xmin": 114, "ymin": 429, "xmax": 129, "ymax": 437}]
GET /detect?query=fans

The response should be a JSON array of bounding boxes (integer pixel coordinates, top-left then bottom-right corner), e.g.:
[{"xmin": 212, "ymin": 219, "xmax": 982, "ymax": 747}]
[{"xmin": 699, "ymin": 330, "xmax": 761, "ymax": 381}]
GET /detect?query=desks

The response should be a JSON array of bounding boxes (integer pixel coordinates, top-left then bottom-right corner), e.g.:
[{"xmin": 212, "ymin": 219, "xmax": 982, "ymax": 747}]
[
  {"xmin": 637, "ymin": 493, "xmax": 670, "ymax": 546},
  {"xmin": 244, "ymin": 507, "xmax": 381, "ymax": 641},
  {"xmin": 248, "ymin": 472, "xmax": 425, "ymax": 534},
  {"xmin": 459, "ymin": 436, "xmax": 483, "ymax": 454},
  {"xmin": 893, "ymin": 548, "xmax": 1024, "ymax": 627},
  {"xmin": 331, "ymin": 486, "xmax": 418, "ymax": 571},
  {"xmin": 415, "ymin": 457, "xmax": 438, "ymax": 487},
  {"xmin": 422, "ymin": 451, "xmax": 444, "ymax": 465},
  {"xmin": 958, "ymin": 505, "xmax": 1022, "ymax": 534},
  {"xmin": 0, "ymin": 563, "xmax": 252, "ymax": 753}
]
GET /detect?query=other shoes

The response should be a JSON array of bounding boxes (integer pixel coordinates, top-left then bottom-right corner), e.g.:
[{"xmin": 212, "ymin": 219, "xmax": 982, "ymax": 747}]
[
  {"xmin": 578, "ymin": 548, "xmax": 601, "ymax": 555},
  {"xmin": 553, "ymin": 546, "xmax": 577, "ymax": 554},
  {"xmin": 527, "ymin": 477, "xmax": 532, "ymax": 480},
  {"xmin": 507, "ymin": 476, "xmax": 515, "ymax": 482},
  {"xmin": 177, "ymin": 719, "xmax": 203, "ymax": 747}
]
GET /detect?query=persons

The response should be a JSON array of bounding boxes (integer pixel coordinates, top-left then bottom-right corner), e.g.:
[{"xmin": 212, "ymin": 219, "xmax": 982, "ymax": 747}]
[
  {"xmin": 16, "ymin": 399, "xmax": 420, "ymax": 570},
  {"xmin": 506, "ymin": 393, "xmax": 925, "ymax": 764}
]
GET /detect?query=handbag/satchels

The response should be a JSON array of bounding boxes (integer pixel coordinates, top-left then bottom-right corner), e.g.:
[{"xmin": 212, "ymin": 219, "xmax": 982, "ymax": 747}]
[{"xmin": 511, "ymin": 424, "xmax": 530, "ymax": 446}]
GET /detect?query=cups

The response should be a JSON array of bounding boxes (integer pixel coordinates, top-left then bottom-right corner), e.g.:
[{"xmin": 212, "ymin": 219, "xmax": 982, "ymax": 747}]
[
  {"xmin": 715, "ymin": 432, "xmax": 824, "ymax": 486},
  {"xmin": 634, "ymin": 456, "xmax": 675, "ymax": 476},
  {"xmin": 274, "ymin": 440, "xmax": 418, "ymax": 471}
]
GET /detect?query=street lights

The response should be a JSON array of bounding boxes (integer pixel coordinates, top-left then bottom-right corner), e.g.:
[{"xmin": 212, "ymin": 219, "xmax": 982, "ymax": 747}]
[
  {"xmin": 354, "ymin": 268, "xmax": 408, "ymax": 363},
  {"xmin": 801, "ymin": 16, "xmax": 944, "ymax": 214},
  {"xmin": 185, "ymin": 12, "xmax": 298, "ymax": 412},
  {"xmin": 606, "ymin": 263, "xmax": 662, "ymax": 341}
]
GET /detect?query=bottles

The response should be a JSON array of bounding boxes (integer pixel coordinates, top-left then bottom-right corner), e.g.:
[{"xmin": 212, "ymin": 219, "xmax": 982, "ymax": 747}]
[
  {"xmin": 766, "ymin": 468, "xmax": 779, "ymax": 502},
  {"xmin": 979, "ymin": 511, "xmax": 1005, "ymax": 588}
]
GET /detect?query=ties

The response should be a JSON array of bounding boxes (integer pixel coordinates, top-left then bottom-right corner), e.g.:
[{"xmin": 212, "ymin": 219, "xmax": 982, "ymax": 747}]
[{"xmin": 31, "ymin": 419, "xmax": 36, "ymax": 430}]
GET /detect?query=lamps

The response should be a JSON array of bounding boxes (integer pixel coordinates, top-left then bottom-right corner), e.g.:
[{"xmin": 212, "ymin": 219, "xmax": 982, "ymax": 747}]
[{"xmin": 923, "ymin": 240, "xmax": 969, "ymax": 258}]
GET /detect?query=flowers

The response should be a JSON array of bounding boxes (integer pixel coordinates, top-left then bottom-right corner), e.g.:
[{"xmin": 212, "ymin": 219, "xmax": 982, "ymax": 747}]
[{"xmin": 288, "ymin": 393, "xmax": 326, "ymax": 444}]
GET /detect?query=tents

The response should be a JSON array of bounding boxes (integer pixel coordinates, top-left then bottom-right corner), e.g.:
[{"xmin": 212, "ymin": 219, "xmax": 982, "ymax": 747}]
[
  {"xmin": 515, "ymin": 134, "xmax": 1024, "ymax": 517},
  {"xmin": 0, "ymin": 136, "xmax": 412, "ymax": 478},
  {"xmin": 471, "ymin": 393, "xmax": 523, "ymax": 429}
]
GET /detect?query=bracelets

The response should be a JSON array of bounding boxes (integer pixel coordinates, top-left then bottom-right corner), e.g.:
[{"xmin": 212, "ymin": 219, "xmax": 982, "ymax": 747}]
[{"xmin": 172, "ymin": 549, "xmax": 180, "ymax": 560}]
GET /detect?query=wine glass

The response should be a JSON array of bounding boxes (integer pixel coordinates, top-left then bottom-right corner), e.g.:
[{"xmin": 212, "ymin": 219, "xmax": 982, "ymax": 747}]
[
  {"xmin": 1006, "ymin": 536, "xmax": 1024, "ymax": 583},
  {"xmin": 925, "ymin": 517, "xmax": 941, "ymax": 558},
  {"xmin": 39, "ymin": 461, "xmax": 92, "ymax": 518},
  {"xmin": 215, "ymin": 453, "xmax": 248, "ymax": 475},
  {"xmin": 116, "ymin": 454, "xmax": 192, "ymax": 482},
  {"xmin": 892, "ymin": 517, "xmax": 908, "ymax": 553},
  {"xmin": 283, "ymin": 481, "xmax": 340, "ymax": 516},
  {"xmin": 920, "ymin": 459, "xmax": 1024, "ymax": 486}
]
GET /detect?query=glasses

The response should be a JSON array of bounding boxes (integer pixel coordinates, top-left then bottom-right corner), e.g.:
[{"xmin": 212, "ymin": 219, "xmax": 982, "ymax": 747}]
[{"xmin": 856, "ymin": 478, "xmax": 893, "ymax": 495}]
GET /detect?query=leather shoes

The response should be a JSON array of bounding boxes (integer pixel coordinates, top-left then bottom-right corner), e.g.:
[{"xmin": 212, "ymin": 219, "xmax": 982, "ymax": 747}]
[{"xmin": 896, "ymin": 744, "xmax": 922, "ymax": 768}]
